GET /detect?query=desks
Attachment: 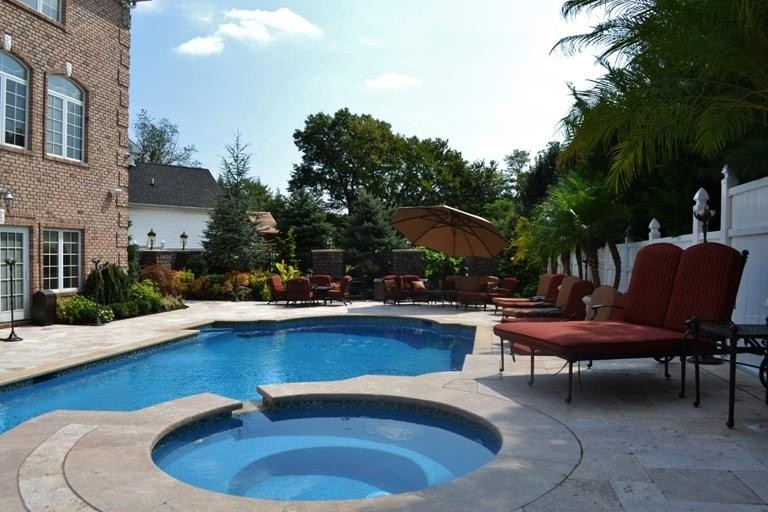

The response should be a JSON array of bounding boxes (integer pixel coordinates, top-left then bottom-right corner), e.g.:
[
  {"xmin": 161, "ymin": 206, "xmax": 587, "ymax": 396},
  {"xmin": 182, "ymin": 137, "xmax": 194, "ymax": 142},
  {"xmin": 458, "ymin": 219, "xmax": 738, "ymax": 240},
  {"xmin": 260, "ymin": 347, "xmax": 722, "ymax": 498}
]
[{"xmin": 684, "ymin": 317, "xmax": 768, "ymax": 428}]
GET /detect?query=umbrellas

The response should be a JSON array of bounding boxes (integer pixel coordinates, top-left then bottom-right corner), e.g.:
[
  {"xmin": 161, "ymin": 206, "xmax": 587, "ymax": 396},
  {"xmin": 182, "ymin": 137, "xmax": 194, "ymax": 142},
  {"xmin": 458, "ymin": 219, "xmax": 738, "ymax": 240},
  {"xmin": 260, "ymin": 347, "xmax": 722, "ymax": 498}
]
[{"xmin": 389, "ymin": 204, "xmax": 512, "ymax": 307}]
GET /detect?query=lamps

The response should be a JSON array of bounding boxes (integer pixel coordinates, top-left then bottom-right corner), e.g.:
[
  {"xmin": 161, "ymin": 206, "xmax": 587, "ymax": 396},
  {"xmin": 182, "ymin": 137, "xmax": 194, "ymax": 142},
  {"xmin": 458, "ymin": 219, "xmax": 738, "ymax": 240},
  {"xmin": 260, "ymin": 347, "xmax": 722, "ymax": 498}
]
[
  {"xmin": 147, "ymin": 228, "xmax": 156, "ymax": 249},
  {"xmin": 0, "ymin": 188, "xmax": 14, "ymax": 217},
  {"xmin": 179, "ymin": 231, "xmax": 188, "ymax": 250},
  {"xmin": 110, "ymin": 188, "xmax": 122, "ymax": 197}
]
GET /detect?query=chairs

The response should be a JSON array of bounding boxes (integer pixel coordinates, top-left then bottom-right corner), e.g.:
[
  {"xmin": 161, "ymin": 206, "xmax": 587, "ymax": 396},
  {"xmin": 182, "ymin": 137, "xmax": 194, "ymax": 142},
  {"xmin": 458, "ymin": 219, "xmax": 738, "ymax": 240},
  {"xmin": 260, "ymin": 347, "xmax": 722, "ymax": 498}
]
[{"xmin": 267, "ymin": 273, "xmax": 352, "ymax": 307}]
